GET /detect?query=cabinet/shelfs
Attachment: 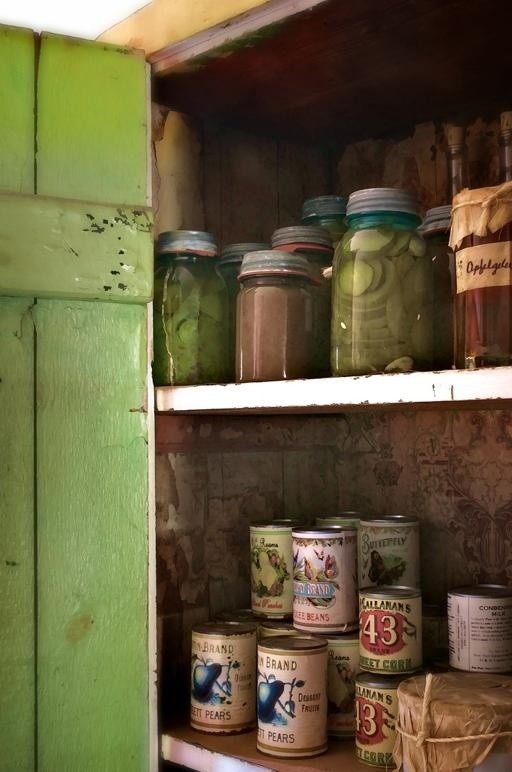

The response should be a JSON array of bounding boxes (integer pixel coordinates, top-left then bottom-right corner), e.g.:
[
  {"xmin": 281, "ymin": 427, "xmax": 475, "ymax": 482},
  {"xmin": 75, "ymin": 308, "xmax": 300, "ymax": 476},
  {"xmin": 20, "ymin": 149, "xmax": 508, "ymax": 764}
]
[{"xmin": 0, "ymin": 0, "xmax": 512, "ymax": 770}]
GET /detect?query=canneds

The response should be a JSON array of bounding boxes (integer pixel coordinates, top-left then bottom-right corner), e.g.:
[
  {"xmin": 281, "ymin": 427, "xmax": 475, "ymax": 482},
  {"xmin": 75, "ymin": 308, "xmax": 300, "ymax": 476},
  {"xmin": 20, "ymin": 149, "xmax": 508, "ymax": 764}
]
[
  {"xmin": 417, "ymin": 202, "xmax": 453, "ymax": 370},
  {"xmin": 446, "ymin": 582, "xmax": 510, "ymax": 673},
  {"xmin": 301, "ymin": 194, "xmax": 346, "ymax": 250},
  {"xmin": 236, "ymin": 249, "xmax": 313, "ymax": 384},
  {"xmin": 153, "ymin": 226, "xmax": 229, "ymax": 386},
  {"xmin": 329, "ymin": 187, "xmax": 436, "ymax": 376},
  {"xmin": 219, "ymin": 240, "xmax": 270, "ymax": 381},
  {"xmin": 191, "ymin": 511, "xmax": 447, "ymax": 769},
  {"xmin": 270, "ymin": 225, "xmax": 333, "ymax": 377}
]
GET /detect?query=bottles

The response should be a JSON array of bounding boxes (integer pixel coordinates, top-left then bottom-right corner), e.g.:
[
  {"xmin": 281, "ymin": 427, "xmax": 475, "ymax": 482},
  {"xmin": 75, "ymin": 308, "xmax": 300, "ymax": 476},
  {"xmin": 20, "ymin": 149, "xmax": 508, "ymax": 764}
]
[
  {"xmin": 153, "ymin": 187, "xmax": 438, "ymax": 387},
  {"xmin": 439, "ymin": 111, "xmax": 511, "ymax": 373}
]
